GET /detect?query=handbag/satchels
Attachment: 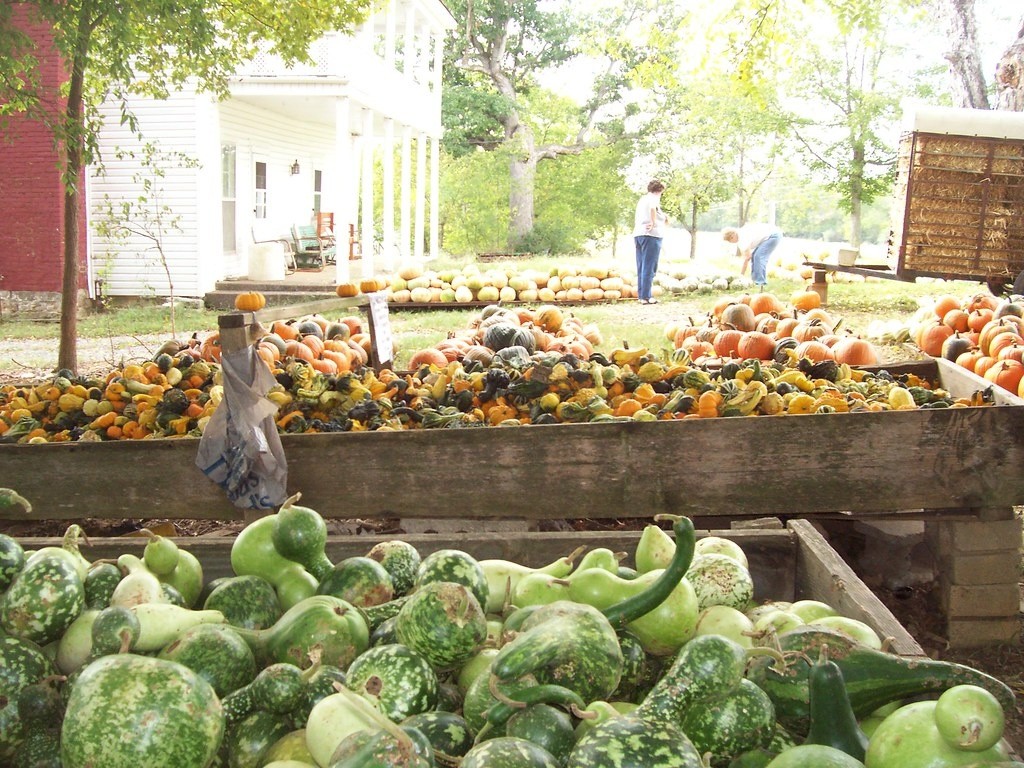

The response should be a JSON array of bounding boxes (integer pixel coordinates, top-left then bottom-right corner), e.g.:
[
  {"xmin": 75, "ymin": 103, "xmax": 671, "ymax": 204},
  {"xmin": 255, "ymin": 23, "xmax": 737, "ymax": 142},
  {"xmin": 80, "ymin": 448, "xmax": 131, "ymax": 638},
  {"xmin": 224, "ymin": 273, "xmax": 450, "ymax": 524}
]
[{"xmin": 195, "ymin": 346, "xmax": 289, "ymax": 510}]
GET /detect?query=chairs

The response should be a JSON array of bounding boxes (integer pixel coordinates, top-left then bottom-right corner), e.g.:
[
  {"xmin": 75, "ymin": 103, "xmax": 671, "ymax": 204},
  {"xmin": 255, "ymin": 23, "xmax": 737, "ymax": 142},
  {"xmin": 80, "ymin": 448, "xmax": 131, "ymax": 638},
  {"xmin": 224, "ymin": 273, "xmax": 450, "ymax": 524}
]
[
  {"xmin": 253, "ymin": 226, "xmax": 297, "ymax": 275},
  {"xmin": 290, "ymin": 222, "xmax": 336, "ymax": 272},
  {"xmin": 317, "ymin": 212, "xmax": 354, "ymax": 260}
]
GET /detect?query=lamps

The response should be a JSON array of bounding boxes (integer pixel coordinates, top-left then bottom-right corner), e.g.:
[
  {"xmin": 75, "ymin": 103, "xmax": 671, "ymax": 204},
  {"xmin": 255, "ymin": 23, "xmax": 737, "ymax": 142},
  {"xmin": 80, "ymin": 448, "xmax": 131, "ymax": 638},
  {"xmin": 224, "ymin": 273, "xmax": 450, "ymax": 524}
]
[{"xmin": 289, "ymin": 159, "xmax": 299, "ymax": 177}]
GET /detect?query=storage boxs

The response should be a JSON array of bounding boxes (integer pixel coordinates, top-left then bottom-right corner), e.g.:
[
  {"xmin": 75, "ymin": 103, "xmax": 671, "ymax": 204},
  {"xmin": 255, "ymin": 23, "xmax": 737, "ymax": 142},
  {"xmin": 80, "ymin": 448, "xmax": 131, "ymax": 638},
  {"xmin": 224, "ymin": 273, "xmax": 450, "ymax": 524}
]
[
  {"xmin": 11, "ymin": 519, "xmax": 1024, "ymax": 768},
  {"xmin": 0, "ymin": 358, "xmax": 1023, "ymax": 521}
]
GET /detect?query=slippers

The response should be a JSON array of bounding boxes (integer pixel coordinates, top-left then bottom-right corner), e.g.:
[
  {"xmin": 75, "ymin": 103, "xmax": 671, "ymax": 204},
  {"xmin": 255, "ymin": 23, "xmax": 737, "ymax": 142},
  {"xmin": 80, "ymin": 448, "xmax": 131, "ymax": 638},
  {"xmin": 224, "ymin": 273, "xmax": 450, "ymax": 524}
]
[{"xmin": 638, "ymin": 297, "xmax": 657, "ymax": 305}]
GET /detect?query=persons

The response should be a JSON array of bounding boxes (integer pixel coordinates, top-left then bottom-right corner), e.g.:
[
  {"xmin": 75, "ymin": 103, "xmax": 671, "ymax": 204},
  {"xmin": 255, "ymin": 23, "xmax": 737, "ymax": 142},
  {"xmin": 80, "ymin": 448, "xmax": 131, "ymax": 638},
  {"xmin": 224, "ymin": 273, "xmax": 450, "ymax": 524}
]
[
  {"xmin": 723, "ymin": 223, "xmax": 782, "ymax": 287},
  {"xmin": 633, "ymin": 180, "xmax": 669, "ymax": 305}
]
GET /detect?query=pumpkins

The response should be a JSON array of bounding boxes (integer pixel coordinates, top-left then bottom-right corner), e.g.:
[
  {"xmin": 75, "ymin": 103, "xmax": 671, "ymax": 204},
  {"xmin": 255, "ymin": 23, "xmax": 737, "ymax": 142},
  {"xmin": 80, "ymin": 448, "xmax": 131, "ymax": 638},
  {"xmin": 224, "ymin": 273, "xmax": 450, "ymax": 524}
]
[{"xmin": 0, "ymin": 265, "xmax": 1024, "ymax": 443}]
